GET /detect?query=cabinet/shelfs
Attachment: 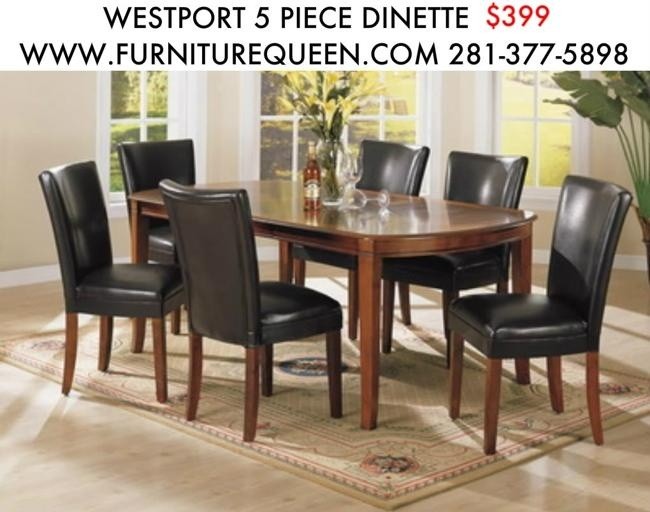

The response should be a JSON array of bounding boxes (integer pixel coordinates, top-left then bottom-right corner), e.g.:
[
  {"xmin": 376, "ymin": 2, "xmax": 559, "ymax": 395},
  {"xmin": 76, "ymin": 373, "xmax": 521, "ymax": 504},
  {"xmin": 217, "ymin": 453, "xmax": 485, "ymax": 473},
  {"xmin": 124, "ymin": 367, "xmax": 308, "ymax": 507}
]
[{"xmin": 302, "ymin": 140, "xmax": 322, "ymax": 213}]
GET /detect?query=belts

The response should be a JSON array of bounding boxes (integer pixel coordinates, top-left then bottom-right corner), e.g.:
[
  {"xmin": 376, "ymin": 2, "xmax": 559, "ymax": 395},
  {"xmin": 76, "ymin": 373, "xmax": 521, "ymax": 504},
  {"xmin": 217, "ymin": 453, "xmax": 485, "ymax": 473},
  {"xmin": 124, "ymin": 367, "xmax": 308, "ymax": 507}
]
[
  {"xmin": 336, "ymin": 152, "xmax": 362, "ymax": 205},
  {"xmin": 342, "ymin": 187, "xmax": 391, "ymax": 211}
]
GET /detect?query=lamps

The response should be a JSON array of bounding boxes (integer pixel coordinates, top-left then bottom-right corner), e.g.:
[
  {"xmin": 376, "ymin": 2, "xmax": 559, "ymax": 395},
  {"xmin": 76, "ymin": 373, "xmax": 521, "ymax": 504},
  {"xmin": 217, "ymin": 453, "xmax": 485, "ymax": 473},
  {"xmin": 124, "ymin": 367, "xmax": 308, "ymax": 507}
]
[
  {"xmin": 380, "ymin": 152, "xmax": 528, "ymax": 367},
  {"xmin": 116, "ymin": 138, "xmax": 196, "ymax": 333},
  {"xmin": 37, "ymin": 157, "xmax": 187, "ymax": 403},
  {"xmin": 293, "ymin": 140, "xmax": 429, "ymax": 341},
  {"xmin": 447, "ymin": 176, "xmax": 634, "ymax": 455},
  {"xmin": 160, "ymin": 178, "xmax": 345, "ymax": 442}
]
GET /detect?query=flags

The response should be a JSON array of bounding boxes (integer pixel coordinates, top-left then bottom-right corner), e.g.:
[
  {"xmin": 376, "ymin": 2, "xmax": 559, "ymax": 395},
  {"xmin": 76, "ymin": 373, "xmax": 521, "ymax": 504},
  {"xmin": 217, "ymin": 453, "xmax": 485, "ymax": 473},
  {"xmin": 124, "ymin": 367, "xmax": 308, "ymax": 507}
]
[{"xmin": 314, "ymin": 140, "xmax": 348, "ymax": 206}]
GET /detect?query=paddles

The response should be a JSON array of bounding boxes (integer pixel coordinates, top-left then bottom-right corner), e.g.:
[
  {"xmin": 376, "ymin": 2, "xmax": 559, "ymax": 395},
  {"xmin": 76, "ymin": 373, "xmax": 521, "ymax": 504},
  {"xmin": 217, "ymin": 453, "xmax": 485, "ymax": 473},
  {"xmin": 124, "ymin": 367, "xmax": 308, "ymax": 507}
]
[{"xmin": 0, "ymin": 320, "xmax": 650, "ymax": 509}]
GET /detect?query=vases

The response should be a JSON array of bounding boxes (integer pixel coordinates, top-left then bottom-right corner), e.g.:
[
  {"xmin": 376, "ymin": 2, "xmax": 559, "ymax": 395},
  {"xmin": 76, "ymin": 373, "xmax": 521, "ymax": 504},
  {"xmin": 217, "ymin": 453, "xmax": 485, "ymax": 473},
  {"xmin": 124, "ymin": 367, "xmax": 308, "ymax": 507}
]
[{"xmin": 271, "ymin": 71, "xmax": 381, "ymax": 197}]
